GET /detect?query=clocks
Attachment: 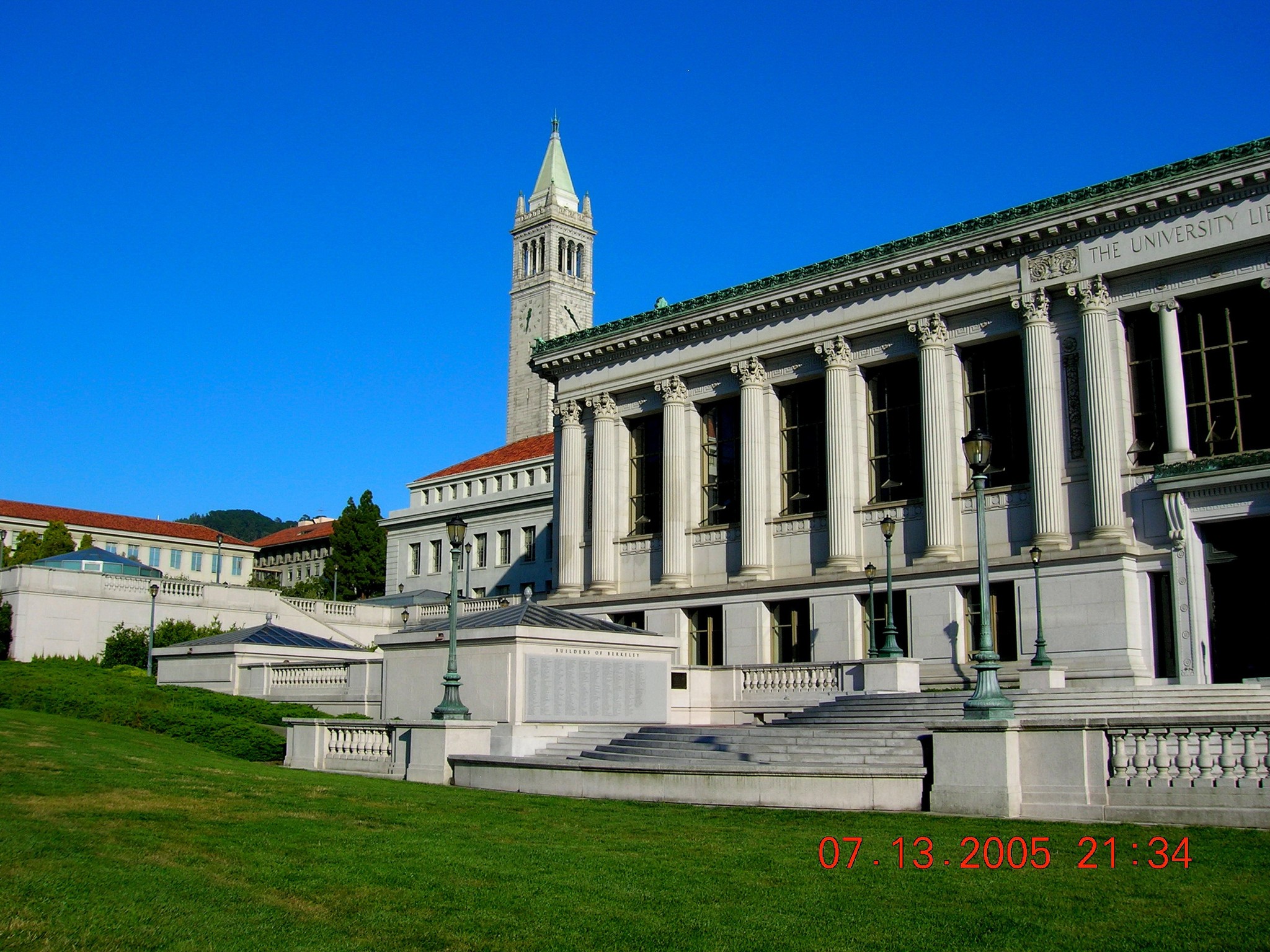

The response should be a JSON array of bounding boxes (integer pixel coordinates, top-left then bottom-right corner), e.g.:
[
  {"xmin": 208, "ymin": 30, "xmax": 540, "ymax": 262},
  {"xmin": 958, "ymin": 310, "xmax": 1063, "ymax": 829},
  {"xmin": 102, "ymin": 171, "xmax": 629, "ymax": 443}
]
[
  {"xmin": 519, "ymin": 299, "xmax": 543, "ymax": 334},
  {"xmin": 558, "ymin": 299, "xmax": 583, "ymax": 334}
]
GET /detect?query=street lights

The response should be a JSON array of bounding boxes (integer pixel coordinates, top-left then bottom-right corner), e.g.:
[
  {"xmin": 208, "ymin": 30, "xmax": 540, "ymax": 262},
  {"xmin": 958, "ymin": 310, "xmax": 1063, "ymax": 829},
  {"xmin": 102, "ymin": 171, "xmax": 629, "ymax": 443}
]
[
  {"xmin": 215, "ymin": 534, "xmax": 224, "ymax": 584},
  {"xmin": 960, "ymin": 428, "xmax": 1017, "ymax": 720},
  {"xmin": 400, "ymin": 608, "xmax": 410, "ymax": 631},
  {"xmin": 1028, "ymin": 545, "xmax": 1053, "ymax": 666},
  {"xmin": 864, "ymin": 562, "xmax": 880, "ymax": 659},
  {"xmin": 879, "ymin": 514, "xmax": 905, "ymax": 658},
  {"xmin": 464, "ymin": 542, "xmax": 473, "ymax": 600},
  {"xmin": 333, "ymin": 562, "xmax": 340, "ymax": 600},
  {"xmin": 397, "ymin": 583, "xmax": 404, "ymax": 593},
  {"xmin": 430, "ymin": 514, "xmax": 473, "ymax": 721},
  {"xmin": 145, "ymin": 584, "xmax": 159, "ymax": 676},
  {"xmin": 0, "ymin": 530, "xmax": 8, "ymax": 570}
]
